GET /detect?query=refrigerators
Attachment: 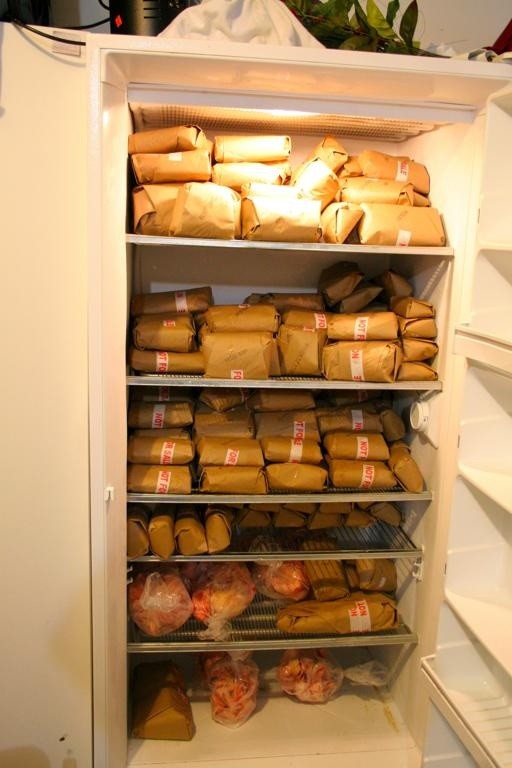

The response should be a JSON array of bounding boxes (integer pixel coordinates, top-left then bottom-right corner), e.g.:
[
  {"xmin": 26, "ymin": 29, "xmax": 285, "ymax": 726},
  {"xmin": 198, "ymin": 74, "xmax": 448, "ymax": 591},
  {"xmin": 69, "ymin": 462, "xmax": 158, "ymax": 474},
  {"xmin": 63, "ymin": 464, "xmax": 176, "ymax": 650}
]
[{"xmin": 84, "ymin": 31, "xmax": 512, "ymax": 767}]
